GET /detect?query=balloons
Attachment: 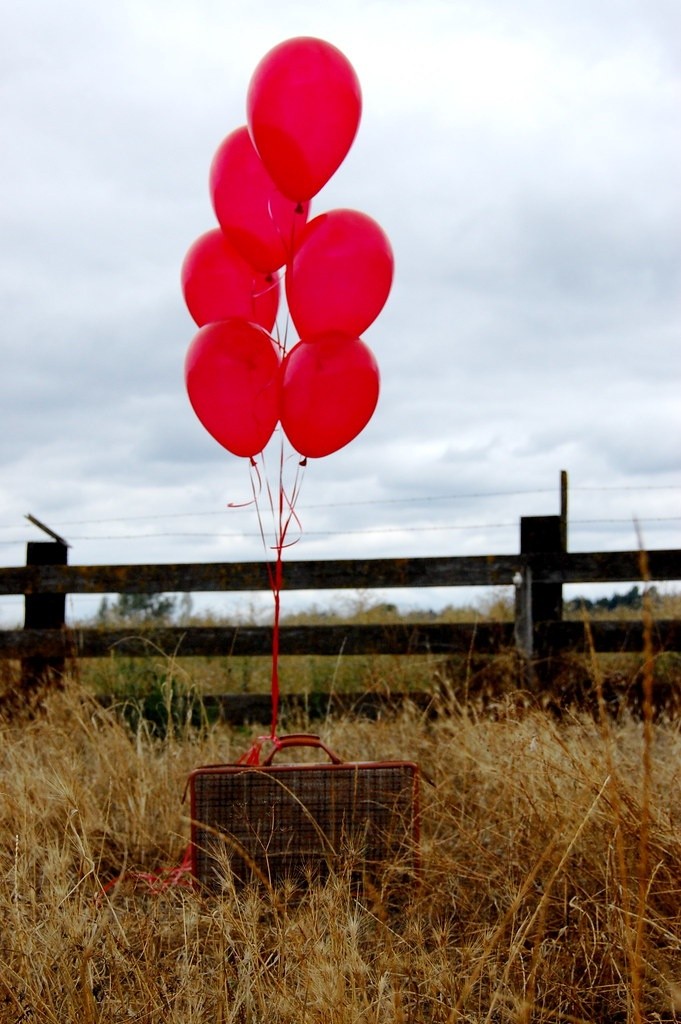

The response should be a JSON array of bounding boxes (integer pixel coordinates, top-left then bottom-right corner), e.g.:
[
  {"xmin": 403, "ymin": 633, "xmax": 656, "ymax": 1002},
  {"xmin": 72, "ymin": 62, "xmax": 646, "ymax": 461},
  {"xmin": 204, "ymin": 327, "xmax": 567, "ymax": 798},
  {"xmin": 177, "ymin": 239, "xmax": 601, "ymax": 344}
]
[
  {"xmin": 245, "ymin": 36, "xmax": 363, "ymax": 214},
  {"xmin": 209, "ymin": 126, "xmax": 311, "ymax": 283},
  {"xmin": 186, "ymin": 321, "xmax": 283, "ymax": 464},
  {"xmin": 280, "ymin": 333, "xmax": 380, "ymax": 465},
  {"xmin": 181, "ymin": 228, "xmax": 281, "ymax": 339},
  {"xmin": 286, "ymin": 210, "xmax": 394, "ymax": 337}
]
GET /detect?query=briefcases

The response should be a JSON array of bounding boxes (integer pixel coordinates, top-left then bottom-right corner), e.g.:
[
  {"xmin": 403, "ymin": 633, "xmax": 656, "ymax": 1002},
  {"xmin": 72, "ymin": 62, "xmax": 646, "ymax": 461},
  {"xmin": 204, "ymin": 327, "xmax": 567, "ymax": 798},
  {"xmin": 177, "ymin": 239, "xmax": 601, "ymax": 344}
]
[{"xmin": 181, "ymin": 734, "xmax": 435, "ymax": 917}]
